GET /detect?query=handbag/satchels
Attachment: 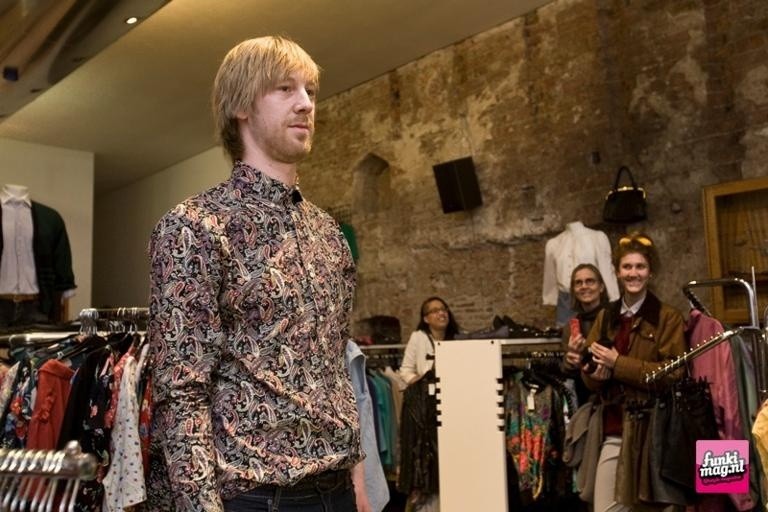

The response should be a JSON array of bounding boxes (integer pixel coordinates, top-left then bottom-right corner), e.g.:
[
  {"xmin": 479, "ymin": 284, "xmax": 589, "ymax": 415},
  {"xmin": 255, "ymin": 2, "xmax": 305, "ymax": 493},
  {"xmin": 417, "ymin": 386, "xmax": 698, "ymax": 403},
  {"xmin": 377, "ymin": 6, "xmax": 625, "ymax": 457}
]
[{"xmin": 603, "ymin": 166, "xmax": 648, "ymax": 223}]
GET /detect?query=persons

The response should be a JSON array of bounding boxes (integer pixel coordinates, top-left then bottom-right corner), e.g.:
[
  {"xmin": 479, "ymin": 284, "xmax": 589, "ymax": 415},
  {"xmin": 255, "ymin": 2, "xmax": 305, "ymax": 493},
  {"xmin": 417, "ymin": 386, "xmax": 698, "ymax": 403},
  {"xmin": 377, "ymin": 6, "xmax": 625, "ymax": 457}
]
[
  {"xmin": 559, "ymin": 264, "xmax": 609, "ymax": 410},
  {"xmin": 146, "ymin": 35, "xmax": 368, "ymax": 510},
  {"xmin": 0, "ymin": 183, "xmax": 78, "ymax": 333},
  {"xmin": 542, "ymin": 220, "xmax": 621, "ymax": 331},
  {"xmin": 397, "ymin": 296, "xmax": 460, "ymax": 512},
  {"xmin": 581, "ymin": 232, "xmax": 687, "ymax": 512}
]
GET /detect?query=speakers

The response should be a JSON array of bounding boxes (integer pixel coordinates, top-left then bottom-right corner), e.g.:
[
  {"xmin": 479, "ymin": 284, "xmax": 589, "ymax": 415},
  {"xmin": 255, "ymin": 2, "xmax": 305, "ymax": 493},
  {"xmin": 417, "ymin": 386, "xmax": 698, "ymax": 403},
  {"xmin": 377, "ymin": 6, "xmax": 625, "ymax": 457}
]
[{"xmin": 432, "ymin": 156, "xmax": 484, "ymax": 214}]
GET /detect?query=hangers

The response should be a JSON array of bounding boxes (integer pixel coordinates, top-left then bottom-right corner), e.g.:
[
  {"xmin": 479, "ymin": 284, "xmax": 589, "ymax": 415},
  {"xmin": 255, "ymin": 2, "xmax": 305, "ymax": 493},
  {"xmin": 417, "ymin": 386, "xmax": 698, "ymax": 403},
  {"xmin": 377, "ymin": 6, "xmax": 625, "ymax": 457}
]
[
  {"xmin": 626, "ymin": 354, "xmax": 710, "ymax": 410},
  {"xmin": 685, "ymin": 299, "xmax": 697, "ymax": 324},
  {"xmin": 0, "ymin": 448, "xmax": 98, "ymax": 512},
  {"xmin": 345, "ymin": 338, "xmax": 404, "ymax": 374},
  {"xmin": 502, "ymin": 351, "xmax": 569, "ymax": 395},
  {"xmin": 420, "ymin": 357, "xmax": 437, "ymax": 385},
  {"xmin": 9, "ymin": 307, "xmax": 159, "ymax": 367}
]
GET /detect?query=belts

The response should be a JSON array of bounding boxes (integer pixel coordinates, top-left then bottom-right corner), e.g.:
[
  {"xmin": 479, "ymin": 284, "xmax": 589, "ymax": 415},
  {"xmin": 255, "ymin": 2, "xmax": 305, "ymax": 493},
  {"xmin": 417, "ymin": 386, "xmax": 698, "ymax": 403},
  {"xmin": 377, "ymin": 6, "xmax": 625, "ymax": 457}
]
[{"xmin": 1, "ymin": 295, "xmax": 35, "ymax": 302}]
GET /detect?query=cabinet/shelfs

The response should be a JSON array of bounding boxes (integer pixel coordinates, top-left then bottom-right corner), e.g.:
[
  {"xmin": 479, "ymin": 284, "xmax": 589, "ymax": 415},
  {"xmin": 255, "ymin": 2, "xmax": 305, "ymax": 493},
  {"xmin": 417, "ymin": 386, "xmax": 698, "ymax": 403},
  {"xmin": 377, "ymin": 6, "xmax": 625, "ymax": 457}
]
[{"xmin": 700, "ymin": 177, "xmax": 768, "ymax": 330}]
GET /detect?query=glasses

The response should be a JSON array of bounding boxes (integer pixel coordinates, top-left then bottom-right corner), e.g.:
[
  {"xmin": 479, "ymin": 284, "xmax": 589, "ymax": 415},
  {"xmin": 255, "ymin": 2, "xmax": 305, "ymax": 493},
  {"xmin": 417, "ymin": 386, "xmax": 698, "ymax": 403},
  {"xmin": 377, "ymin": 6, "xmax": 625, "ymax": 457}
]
[
  {"xmin": 618, "ymin": 235, "xmax": 652, "ymax": 247},
  {"xmin": 575, "ymin": 279, "xmax": 598, "ymax": 287},
  {"xmin": 427, "ymin": 306, "xmax": 446, "ymax": 314}
]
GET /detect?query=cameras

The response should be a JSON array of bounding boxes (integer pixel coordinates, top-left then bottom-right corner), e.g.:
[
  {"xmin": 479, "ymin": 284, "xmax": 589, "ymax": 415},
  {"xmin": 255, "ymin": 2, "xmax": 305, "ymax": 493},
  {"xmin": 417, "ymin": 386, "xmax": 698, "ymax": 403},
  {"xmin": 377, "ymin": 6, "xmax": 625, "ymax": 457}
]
[{"xmin": 580, "ymin": 334, "xmax": 614, "ymax": 372}]
